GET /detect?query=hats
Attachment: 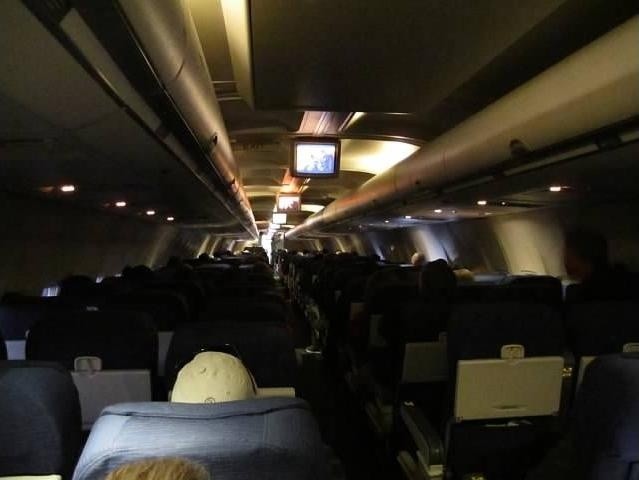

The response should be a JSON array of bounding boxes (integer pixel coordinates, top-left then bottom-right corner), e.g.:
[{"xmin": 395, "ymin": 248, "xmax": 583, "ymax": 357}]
[{"xmin": 171, "ymin": 351, "xmax": 258, "ymax": 403}]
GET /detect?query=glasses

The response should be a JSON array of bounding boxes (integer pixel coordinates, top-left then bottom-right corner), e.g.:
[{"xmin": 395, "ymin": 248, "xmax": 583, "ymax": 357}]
[{"xmin": 171, "ymin": 342, "xmax": 257, "ymax": 394}]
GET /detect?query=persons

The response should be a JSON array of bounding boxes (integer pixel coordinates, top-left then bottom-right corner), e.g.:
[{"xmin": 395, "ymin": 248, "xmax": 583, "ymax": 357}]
[
  {"xmin": 59, "ymin": 244, "xmax": 271, "ymax": 404},
  {"xmin": 275, "ymin": 247, "xmax": 474, "ymax": 296},
  {"xmin": 105, "ymin": 456, "xmax": 211, "ymax": 480}
]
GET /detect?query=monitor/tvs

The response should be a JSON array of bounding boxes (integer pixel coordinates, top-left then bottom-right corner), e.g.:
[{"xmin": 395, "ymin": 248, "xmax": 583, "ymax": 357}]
[
  {"xmin": 266, "ymin": 193, "xmax": 301, "ymax": 239},
  {"xmin": 289, "ymin": 138, "xmax": 342, "ymax": 178}
]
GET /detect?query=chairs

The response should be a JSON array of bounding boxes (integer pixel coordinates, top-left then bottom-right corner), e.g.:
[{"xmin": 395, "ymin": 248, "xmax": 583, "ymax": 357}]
[
  {"xmin": 275, "ymin": 249, "xmax": 638, "ymax": 480},
  {"xmin": 0, "ymin": 249, "xmax": 339, "ymax": 480}
]
